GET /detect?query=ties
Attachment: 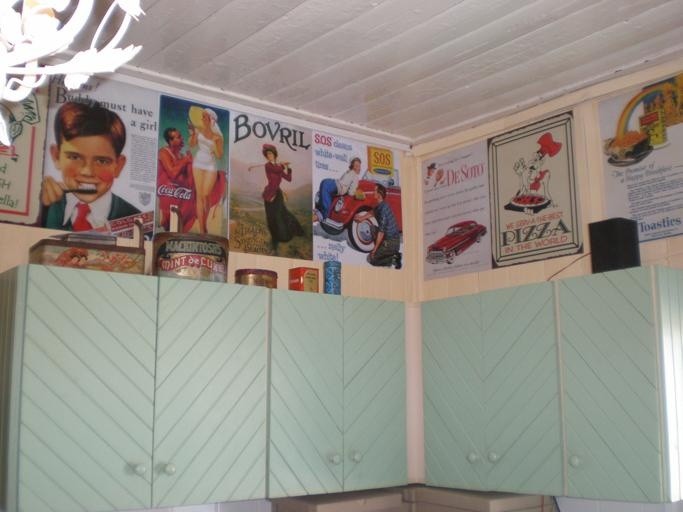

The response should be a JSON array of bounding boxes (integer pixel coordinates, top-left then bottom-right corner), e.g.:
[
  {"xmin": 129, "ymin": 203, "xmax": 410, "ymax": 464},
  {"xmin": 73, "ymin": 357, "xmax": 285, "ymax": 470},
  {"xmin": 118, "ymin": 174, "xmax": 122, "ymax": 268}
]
[{"xmin": 71, "ymin": 202, "xmax": 93, "ymax": 233}]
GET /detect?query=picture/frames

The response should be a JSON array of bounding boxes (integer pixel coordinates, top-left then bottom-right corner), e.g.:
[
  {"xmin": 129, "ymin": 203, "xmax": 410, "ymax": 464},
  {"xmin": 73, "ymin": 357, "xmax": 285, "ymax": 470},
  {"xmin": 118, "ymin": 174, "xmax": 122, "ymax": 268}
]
[{"xmin": 486, "ymin": 104, "xmax": 585, "ymax": 270}]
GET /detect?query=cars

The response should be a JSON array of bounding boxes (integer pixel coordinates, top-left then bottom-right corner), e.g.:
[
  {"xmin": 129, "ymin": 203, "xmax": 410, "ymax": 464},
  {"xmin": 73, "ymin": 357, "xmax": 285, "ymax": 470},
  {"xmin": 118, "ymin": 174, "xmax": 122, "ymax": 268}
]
[
  {"xmin": 425, "ymin": 220, "xmax": 487, "ymax": 265},
  {"xmin": 315, "ymin": 163, "xmax": 403, "ymax": 253}
]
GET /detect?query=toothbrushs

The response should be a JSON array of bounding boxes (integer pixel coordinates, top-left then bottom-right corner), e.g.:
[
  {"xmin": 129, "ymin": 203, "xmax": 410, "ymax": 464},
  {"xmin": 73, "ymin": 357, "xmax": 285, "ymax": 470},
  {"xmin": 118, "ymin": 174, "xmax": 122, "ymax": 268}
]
[{"xmin": 63, "ymin": 182, "xmax": 98, "ymax": 194}]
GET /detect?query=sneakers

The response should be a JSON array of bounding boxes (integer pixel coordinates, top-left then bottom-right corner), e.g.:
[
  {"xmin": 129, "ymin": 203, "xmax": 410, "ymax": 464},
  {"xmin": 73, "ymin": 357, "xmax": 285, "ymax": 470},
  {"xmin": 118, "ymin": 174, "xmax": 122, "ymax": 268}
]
[{"xmin": 392, "ymin": 252, "xmax": 402, "ymax": 269}]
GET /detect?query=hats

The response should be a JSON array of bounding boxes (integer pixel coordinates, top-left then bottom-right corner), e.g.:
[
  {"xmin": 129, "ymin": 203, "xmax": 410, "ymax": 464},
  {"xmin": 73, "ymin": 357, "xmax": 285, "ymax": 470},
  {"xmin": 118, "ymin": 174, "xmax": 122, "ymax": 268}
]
[
  {"xmin": 189, "ymin": 105, "xmax": 224, "ymax": 136},
  {"xmin": 261, "ymin": 144, "xmax": 276, "ymax": 149}
]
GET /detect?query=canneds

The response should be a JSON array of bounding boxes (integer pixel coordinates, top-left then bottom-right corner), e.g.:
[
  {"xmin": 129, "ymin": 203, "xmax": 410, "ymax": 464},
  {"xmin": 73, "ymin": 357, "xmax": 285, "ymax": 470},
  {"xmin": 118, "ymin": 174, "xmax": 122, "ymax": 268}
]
[
  {"xmin": 234, "ymin": 268, "xmax": 277, "ymax": 289},
  {"xmin": 368, "ymin": 144, "xmax": 395, "ymax": 180}
]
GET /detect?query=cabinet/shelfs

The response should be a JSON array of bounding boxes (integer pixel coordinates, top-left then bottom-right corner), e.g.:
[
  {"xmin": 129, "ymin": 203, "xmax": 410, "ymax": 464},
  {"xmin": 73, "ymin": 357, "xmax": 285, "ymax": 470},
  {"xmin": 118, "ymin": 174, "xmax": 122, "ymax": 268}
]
[
  {"xmin": 555, "ymin": 266, "xmax": 683, "ymax": 504},
  {"xmin": 416, "ymin": 278, "xmax": 562, "ymax": 498},
  {"xmin": 270, "ymin": 264, "xmax": 407, "ymax": 502},
  {"xmin": 1, "ymin": 264, "xmax": 269, "ymax": 511}
]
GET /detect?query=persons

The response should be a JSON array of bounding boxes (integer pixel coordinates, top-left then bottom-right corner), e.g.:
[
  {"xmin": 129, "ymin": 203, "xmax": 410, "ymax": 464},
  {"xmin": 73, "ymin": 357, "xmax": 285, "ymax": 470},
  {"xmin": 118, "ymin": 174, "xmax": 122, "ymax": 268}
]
[
  {"xmin": 35, "ymin": 98, "xmax": 150, "ymax": 240},
  {"xmin": 262, "ymin": 142, "xmax": 297, "ymax": 252},
  {"xmin": 315, "ymin": 157, "xmax": 402, "ymax": 267},
  {"xmin": 157, "ymin": 110, "xmax": 227, "ymax": 234}
]
